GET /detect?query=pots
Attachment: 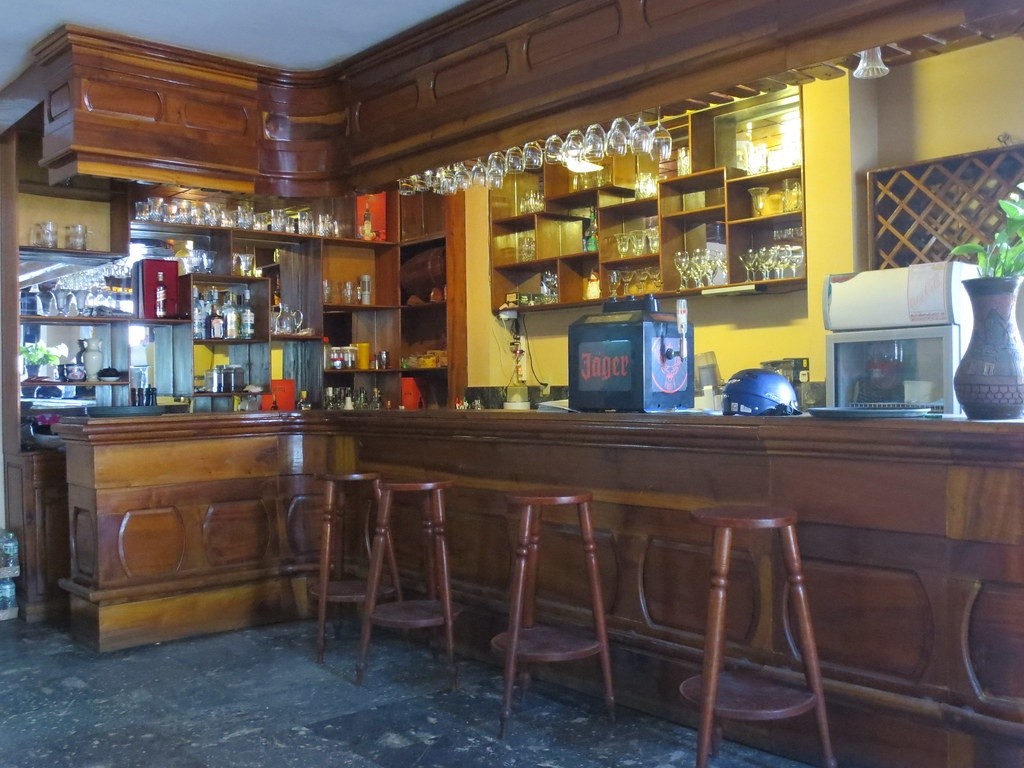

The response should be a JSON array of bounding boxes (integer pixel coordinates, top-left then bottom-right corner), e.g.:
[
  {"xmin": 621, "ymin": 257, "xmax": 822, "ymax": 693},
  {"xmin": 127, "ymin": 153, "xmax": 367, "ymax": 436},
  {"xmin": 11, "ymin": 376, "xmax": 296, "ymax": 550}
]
[{"xmin": 204, "ymin": 364, "xmax": 245, "ymax": 394}]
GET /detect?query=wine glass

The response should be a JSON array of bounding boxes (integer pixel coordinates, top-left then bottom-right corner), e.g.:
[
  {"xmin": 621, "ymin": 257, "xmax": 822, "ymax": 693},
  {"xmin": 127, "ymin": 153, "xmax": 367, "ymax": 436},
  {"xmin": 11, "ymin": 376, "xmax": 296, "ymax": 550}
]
[
  {"xmin": 645, "ymin": 228, "xmax": 659, "ymax": 254},
  {"xmin": 648, "ymin": 106, "xmax": 673, "ymax": 160},
  {"xmin": 606, "ymin": 264, "xmax": 660, "ymax": 298},
  {"xmin": 629, "ymin": 230, "xmax": 647, "ymax": 257},
  {"xmin": 747, "ymin": 186, "xmax": 770, "ymax": 217},
  {"xmin": 738, "ymin": 243, "xmax": 804, "ymax": 283},
  {"xmin": 629, "ymin": 111, "xmax": 651, "ymax": 155},
  {"xmin": 189, "ymin": 249, "xmax": 217, "ymax": 275},
  {"xmin": 674, "ymin": 249, "xmax": 728, "ymax": 290},
  {"xmin": 230, "ymin": 253, "xmax": 254, "ymax": 276},
  {"xmin": 543, "ymin": 265, "xmax": 558, "ymax": 303},
  {"xmin": 614, "ymin": 233, "xmax": 630, "ymax": 258},
  {"xmin": 470, "ymin": 156, "xmax": 487, "ymax": 187}
]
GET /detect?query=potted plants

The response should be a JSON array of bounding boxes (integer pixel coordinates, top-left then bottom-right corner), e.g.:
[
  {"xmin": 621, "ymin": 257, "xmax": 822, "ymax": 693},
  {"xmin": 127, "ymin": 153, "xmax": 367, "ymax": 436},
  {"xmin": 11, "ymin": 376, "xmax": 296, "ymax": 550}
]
[{"xmin": 947, "ymin": 183, "xmax": 1023, "ymax": 418}]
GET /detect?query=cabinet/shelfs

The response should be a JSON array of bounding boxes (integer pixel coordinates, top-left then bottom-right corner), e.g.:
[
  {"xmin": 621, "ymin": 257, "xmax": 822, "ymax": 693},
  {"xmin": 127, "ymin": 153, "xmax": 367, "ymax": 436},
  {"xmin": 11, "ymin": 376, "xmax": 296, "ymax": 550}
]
[
  {"xmin": 15, "ymin": 176, "xmax": 448, "ymax": 415},
  {"xmin": 484, "ymin": 79, "xmax": 800, "ymax": 314}
]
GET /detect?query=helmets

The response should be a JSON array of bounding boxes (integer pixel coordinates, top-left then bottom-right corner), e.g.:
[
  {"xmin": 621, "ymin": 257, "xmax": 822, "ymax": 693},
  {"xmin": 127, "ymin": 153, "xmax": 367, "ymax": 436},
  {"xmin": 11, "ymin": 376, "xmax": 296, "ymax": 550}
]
[{"xmin": 720, "ymin": 368, "xmax": 803, "ymax": 416}]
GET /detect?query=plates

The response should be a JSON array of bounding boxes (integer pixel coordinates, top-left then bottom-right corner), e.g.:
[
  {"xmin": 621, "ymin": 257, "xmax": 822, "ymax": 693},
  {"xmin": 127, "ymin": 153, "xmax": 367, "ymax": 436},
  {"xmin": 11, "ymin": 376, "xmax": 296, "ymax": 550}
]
[
  {"xmin": 97, "ymin": 376, "xmax": 121, "ymax": 382},
  {"xmin": 85, "ymin": 405, "xmax": 166, "ymax": 417}
]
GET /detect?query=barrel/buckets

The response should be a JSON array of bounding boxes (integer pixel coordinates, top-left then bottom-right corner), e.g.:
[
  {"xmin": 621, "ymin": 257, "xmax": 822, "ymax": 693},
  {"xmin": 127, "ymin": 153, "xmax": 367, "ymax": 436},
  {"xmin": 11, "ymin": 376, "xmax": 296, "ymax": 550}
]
[{"xmin": 401, "ymin": 246, "xmax": 446, "ymax": 298}]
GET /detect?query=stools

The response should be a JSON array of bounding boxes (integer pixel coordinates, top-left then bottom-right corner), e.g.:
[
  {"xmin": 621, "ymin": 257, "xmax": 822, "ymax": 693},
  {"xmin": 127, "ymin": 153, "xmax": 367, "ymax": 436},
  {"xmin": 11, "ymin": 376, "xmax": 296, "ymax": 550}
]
[
  {"xmin": 675, "ymin": 501, "xmax": 835, "ymax": 767},
  {"xmin": 490, "ymin": 488, "xmax": 614, "ymax": 715},
  {"xmin": 351, "ymin": 479, "xmax": 463, "ymax": 694},
  {"xmin": 305, "ymin": 470, "xmax": 413, "ymax": 665}
]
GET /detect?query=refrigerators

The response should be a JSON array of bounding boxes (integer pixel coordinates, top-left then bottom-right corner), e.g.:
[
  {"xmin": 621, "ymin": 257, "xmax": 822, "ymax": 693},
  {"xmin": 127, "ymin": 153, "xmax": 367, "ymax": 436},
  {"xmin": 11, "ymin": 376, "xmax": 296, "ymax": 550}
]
[{"xmin": 823, "ymin": 261, "xmax": 982, "ymax": 417}]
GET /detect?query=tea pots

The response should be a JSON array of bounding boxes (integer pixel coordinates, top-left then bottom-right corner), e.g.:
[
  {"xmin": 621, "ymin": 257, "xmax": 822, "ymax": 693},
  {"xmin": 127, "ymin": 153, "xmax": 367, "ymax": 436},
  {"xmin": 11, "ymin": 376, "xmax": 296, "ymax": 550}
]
[{"xmin": 275, "ymin": 303, "xmax": 304, "ymax": 335}]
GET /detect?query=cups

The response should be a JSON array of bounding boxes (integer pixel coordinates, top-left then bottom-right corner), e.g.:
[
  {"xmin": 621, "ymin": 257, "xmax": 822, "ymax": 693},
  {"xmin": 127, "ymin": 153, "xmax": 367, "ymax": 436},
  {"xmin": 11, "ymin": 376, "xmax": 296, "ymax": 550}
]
[
  {"xmin": 676, "ymin": 147, "xmax": 691, "ymax": 176},
  {"xmin": 323, "ymin": 278, "xmax": 361, "ymax": 305},
  {"xmin": 350, "ymin": 343, "xmax": 369, "ymax": 369},
  {"xmin": 518, "ymin": 237, "xmax": 534, "ymax": 262},
  {"xmin": 903, "ymin": 380, "xmax": 933, "ymax": 403},
  {"xmin": 520, "ymin": 191, "xmax": 544, "ymax": 214},
  {"xmin": 396, "ymin": 115, "xmax": 631, "ymax": 196},
  {"xmin": 64, "ymin": 364, "xmax": 85, "ymax": 381},
  {"xmin": 56, "ymin": 257, "xmax": 132, "ymax": 292},
  {"xmin": 113, "ymin": 385, "xmax": 129, "ymax": 406},
  {"xmin": 635, "ymin": 172, "xmax": 667, "ymax": 200},
  {"xmin": 136, "ymin": 195, "xmax": 339, "ymax": 237},
  {"xmin": 781, "ymin": 178, "xmax": 801, "ymax": 212},
  {"xmin": 95, "ymin": 385, "xmax": 112, "ymax": 406},
  {"xmin": 32, "ymin": 221, "xmax": 58, "ymax": 249},
  {"xmin": 65, "ymin": 224, "xmax": 95, "ymax": 250}
]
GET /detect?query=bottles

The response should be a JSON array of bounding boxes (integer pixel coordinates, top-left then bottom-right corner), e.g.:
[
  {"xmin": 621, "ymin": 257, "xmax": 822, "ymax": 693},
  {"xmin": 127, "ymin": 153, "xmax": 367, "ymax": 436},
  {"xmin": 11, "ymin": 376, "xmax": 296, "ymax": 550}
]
[
  {"xmin": 0, "ymin": 530, "xmax": 20, "ymax": 610},
  {"xmin": 193, "ymin": 285, "xmax": 255, "ymax": 340},
  {"xmin": 296, "ymin": 390, "xmax": 311, "ymax": 410},
  {"xmin": 324, "ymin": 336, "xmax": 355, "ymax": 371},
  {"xmin": 81, "ymin": 337, "xmax": 104, "ymax": 381},
  {"xmin": 583, "ymin": 204, "xmax": 598, "ymax": 252},
  {"xmin": 155, "ymin": 271, "xmax": 167, "ymax": 319},
  {"xmin": 145, "ymin": 387, "xmax": 158, "ymax": 405},
  {"xmin": 325, "ymin": 386, "xmax": 392, "ymax": 411},
  {"xmin": 364, "ymin": 201, "xmax": 372, "ymax": 239}
]
